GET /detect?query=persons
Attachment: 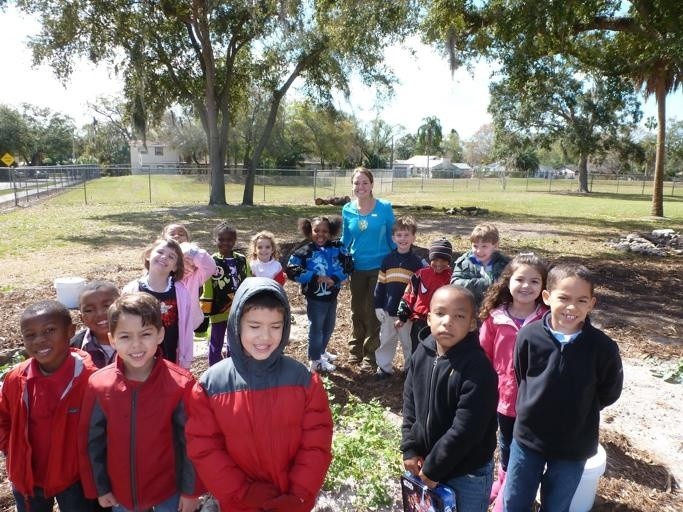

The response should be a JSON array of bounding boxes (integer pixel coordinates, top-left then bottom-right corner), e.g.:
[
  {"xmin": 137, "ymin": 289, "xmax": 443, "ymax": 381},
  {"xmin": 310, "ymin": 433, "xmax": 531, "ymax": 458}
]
[
  {"xmin": 339, "ymin": 167, "xmax": 397, "ymax": 365},
  {"xmin": 194, "ymin": 216, "xmax": 355, "ymax": 372},
  {"xmin": 400, "ymin": 283, "xmax": 500, "ymax": 511},
  {"xmin": 449, "ymin": 222, "xmax": 513, "ymax": 306},
  {"xmin": 501, "ymin": 265, "xmax": 626, "ymax": 512},
  {"xmin": 161, "ymin": 221, "xmax": 218, "ymax": 332},
  {"xmin": 69, "ymin": 279, "xmax": 122, "ymax": 370},
  {"xmin": 0, "ymin": 300, "xmax": 114, "ymax": 512},
  {"xmin": 374, "ymin": 218, "xmax": 426, "ymax": 378},
  {"xmin": 183, "ymin": 277, "xmax": 334, "ymax": 512},
  {"xmin": 392, "ymin": 239, "xmax": 455, "ymax": 353},
  {"xmin": 118, "ymin": 237, "xmax": 194, "ymax": 371},
  {"xmin": 478, "ymin": 250, "xmax": 552, "ymax": 504},
  {"xmin": 73, "ymin": 292, "xmax": 211, "ymax": 511}
]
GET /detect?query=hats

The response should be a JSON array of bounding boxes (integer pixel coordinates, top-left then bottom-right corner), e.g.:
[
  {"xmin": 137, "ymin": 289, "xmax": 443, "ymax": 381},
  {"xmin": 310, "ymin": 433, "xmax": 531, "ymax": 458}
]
[{"xmin": 429, "ymin": 240, "xmax": 453, "ymax": 263}]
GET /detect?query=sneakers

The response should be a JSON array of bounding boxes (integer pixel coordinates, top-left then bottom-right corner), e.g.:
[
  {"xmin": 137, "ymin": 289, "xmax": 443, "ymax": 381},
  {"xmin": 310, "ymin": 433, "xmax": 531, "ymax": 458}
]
[
  {"xmin": 371, "ymin": 370, "xmax": 387, "ymax": 382},
  {"xmin": 347, "ymin": 353, "xmax": 363, "ymax": 363},
  {"xmin": 311, "ymin": 361, "xmax": 338, "ymax": 372},
  {"xmin": 322, "ymin": 351, "xmax": 336, "ymax": 361}
]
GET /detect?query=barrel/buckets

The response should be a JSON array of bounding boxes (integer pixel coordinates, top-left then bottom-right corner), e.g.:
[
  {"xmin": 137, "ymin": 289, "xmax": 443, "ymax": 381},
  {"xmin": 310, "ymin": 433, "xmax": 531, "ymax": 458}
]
[
  {"xmin": 53, "ymin": 277, "xmax": 87, "ymax": 308},
  {"xmin": 534, "ymin": 443, "xmax": 607, "ymax": 511}
]
